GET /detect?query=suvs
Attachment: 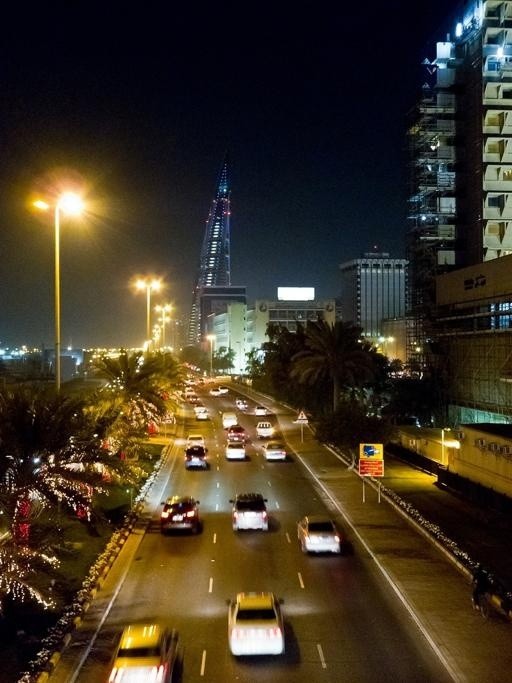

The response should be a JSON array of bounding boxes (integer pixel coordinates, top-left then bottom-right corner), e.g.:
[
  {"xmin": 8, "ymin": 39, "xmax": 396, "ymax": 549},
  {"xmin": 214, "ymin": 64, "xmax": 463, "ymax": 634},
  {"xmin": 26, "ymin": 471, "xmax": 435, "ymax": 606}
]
[
  {"xmin": 160, "ymin": 495, "xmax": 201, "ymax": 535},
  {"xmin": 228, "ymin": 492, "xmax": 270, "ymax": 533}
]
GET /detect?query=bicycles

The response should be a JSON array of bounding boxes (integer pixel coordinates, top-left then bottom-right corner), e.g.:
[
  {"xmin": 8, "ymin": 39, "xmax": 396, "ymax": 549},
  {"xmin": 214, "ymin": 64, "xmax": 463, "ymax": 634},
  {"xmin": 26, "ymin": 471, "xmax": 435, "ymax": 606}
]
[{"xmin": 469, "ymin": 585, "xmax": 492, "ymax": 620}]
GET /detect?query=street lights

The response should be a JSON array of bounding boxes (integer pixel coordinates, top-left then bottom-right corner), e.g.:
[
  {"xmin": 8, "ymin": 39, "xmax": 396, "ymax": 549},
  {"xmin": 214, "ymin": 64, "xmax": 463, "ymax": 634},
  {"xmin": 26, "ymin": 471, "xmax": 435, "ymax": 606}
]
[
  {"xmin": 441, "ymin": 426, "xmax": 451, "ymax": 468},
  {"xmin": 207, "ymin": 333, "xmax": 216, "ymax": 375},
  {"xmin": 152, "ymin": 302, "xmax": 173, "ymax": 353},
  {"xmin": 135, "ymin": 273, "xmax": 163, "ymax": 354},
  {"xmin": 29, "ymin": 164, "xmax": 95, "ymax": 392}
]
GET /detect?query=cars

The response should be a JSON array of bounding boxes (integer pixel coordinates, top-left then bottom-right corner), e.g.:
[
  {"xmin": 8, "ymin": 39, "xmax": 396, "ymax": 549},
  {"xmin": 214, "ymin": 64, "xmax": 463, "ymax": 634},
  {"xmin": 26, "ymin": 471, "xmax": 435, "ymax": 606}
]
[
  {"xmin": 228, "ymin": 591, "xmax": 288, "ymax": 659},
  {"xmin": 180, "ymin": 361, "xmax": 287, "ymax": 469},
  {"xmin": 109, "ymin": 622, "xmax": 178, "ymax": 682},
  {"xmin": 296, "ymin": 512, "xmax": 342, "ymax": 555}
]
